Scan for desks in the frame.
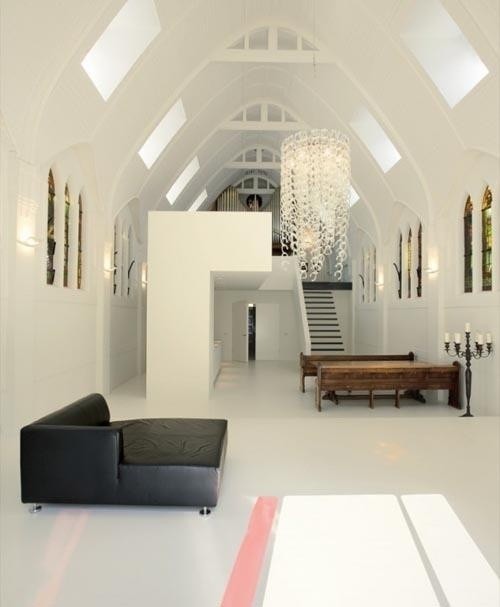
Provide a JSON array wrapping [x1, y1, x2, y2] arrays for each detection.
[[297, 352, 462, 412]]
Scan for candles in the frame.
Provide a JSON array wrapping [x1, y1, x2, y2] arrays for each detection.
[[445, 322, 492, 345]]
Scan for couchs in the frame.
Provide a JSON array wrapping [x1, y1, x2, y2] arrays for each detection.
[[19, 390, 231, 515]]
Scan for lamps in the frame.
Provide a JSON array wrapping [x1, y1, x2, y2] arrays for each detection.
[[276, 0, 355, 282]]
